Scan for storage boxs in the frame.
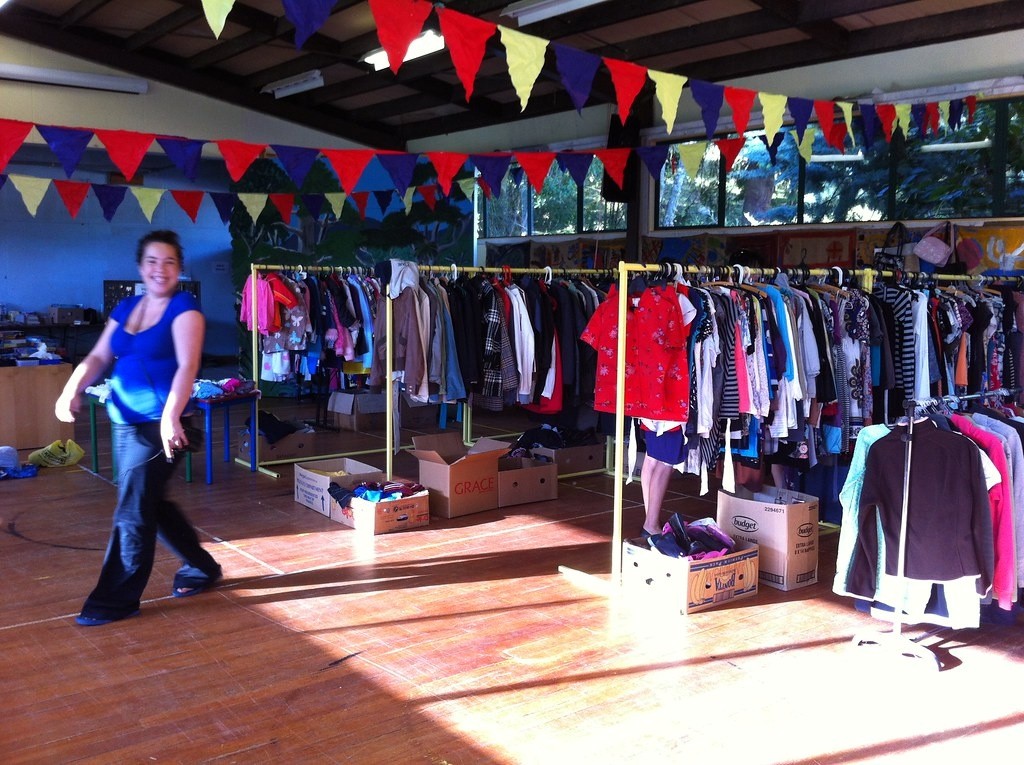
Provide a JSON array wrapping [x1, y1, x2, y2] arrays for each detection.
[[624, 444, 682, 480], [529, 444, 604, 476], [48, 307, 84, 324], [0, 331, 63, 367], [237, 431, 315, 463], [716, 486, 820, 592], [294, 457, 383, 519], [622, 535, 760, 616], [330, 490, 429, 536], [497, 456, 559, 508], [398, 392, 441, 428], [327, 389, 386, 433], [405, 432, 513, 519]]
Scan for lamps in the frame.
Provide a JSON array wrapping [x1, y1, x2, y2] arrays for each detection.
[[810, 153, 865, 162], [0, 63, 149, 96], [921, 139, 994, 152], [259, 70, 324, 100], [499, 0, 607, 28], [358, 27, 445, 73]]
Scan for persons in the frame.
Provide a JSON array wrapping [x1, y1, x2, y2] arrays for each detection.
[[641, 447, 786, 536], [55, 230, 222, 625]]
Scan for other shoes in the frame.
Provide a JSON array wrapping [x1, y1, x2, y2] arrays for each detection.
[[647, 512, 736, 561], [641, 527, 651, 537]]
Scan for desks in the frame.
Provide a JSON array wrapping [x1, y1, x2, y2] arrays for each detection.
[[0, 316, 105, 369], [191, 392, 260, 486], [0, 362, 75, 450], [85, 393, 191, 484]]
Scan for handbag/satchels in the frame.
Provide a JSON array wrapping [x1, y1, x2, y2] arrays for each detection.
[[172, 418, 203, 455], [29, 439, 85, 468]]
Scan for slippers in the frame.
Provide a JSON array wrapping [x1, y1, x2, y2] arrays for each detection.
[[172, 571, 223, 597], [75, 610, 142, 626]]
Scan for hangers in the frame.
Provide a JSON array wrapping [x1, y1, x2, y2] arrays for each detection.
[[626, 263, 1024, 295], [418, 258, 616, 287], [900, 387, 1016, 423], [256, 263, 374, 279]]
